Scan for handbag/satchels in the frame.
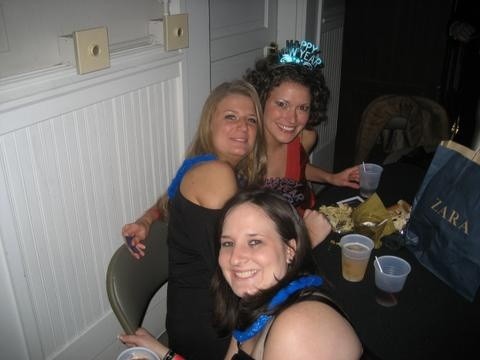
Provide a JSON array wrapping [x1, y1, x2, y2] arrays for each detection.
[[401, 136, 480, 303]]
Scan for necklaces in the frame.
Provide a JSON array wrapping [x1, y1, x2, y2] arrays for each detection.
[[232, 275, 323, 342]]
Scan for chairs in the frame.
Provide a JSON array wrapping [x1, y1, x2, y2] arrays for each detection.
[[355, 95, 448, 166], [106, 219, 169, 348]]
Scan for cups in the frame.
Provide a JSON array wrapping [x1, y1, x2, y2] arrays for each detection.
[[340, 234, 374, 282], [117, 347, 160, 360], [359, 164, 383, 198]]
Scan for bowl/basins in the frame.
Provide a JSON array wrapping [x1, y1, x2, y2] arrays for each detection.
[[373, 256, 411, 292]]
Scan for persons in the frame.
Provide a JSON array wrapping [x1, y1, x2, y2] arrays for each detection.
[[164, 78, 268, 360], [122, 39, 361, 261], [117, 189, 364, 360]]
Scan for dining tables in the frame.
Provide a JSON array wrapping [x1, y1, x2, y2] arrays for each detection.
[[310, 161, 480, 360]]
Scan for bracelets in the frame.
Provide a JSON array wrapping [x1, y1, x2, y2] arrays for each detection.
[[164, 350, 174, 360]]
